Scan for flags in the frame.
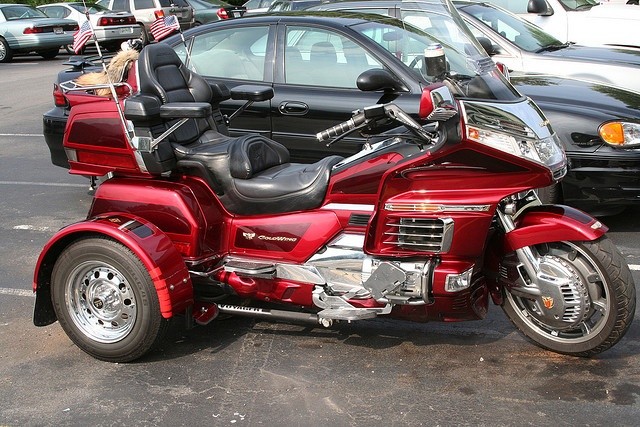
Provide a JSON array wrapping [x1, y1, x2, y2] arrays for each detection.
[[73, 12, 96, 54], [150, 14, 183, 41]]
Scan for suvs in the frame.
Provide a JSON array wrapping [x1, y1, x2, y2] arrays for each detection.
[[85, 0, 196, 48]]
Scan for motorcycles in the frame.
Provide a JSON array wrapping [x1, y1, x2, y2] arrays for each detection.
[[23, 1, 637, 363]]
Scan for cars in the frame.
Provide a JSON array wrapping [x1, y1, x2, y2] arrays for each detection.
[[20, 1, 143, 54], [375, 0, 640, 49], [0, 2, 80, 62], [42, 10, 640, 220], [241, 0, 289, 13], [248, 0, 640, 95], [185, 0, 248, 28]]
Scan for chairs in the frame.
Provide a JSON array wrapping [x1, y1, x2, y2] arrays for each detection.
[[136, 36, 346, 219], [284, 44, 307, 85], [307, 39, 354, 89]]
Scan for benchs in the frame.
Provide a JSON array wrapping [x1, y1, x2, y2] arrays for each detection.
[[195, 48, 265, 83]]
